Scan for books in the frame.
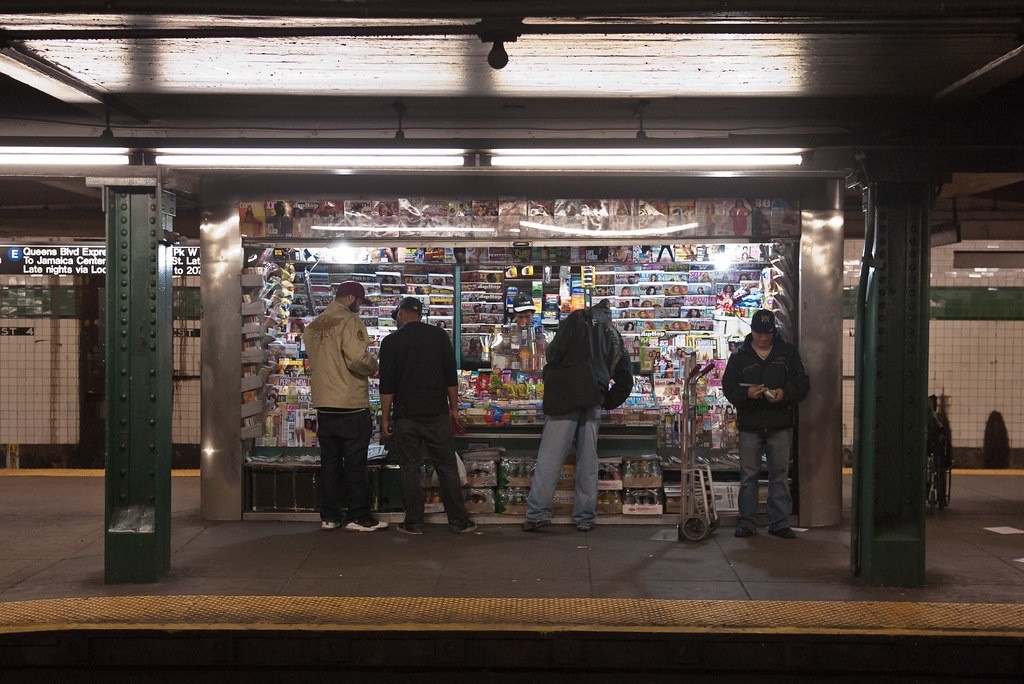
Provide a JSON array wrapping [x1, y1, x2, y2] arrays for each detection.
[[239, 193, 800, 374], [287, 408, 321, 448]]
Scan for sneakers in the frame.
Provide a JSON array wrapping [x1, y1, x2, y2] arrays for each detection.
[[396, 517, 423, 534], [449, 521, 478, 532], [345, 517, 388, 532], [320, 512, 344, 529]]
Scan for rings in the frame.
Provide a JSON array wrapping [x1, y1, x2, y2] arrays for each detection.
[[382, 432, 384, 433]]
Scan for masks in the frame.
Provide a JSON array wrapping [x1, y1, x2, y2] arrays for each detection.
[[348, 297, 360, 313]]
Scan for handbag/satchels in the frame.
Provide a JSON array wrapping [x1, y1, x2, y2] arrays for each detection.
[[542, 362, 600, 416], [431, 450, 468, 488]]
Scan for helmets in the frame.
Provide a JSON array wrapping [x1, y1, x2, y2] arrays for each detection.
[[391, 297, 422, 320]]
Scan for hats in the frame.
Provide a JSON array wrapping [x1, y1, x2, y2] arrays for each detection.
[[593, 299, 612, 319], [335, 281, 373, 304], [513, 292, 537, 312], [751, 309, 775, 333]]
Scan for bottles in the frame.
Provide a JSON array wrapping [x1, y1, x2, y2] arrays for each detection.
[[423, 487, 440, 504], [596, 462, 622, 514], [498, 457, 537, 505], [465, 460, 496, 504], [623, 457, 662, 505], [553, 465, 574, 515]]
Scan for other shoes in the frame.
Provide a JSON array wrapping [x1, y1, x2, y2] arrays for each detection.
[[521, 520, 551, 532], [768, 527, 794, 538], [578, 523, 591, 531], [735, 527, 752, 537]]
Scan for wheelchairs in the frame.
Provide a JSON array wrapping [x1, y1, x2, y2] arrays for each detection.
[[928, 437, 954, 513]]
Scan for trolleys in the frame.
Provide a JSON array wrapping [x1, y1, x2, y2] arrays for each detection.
[[650, 364, 722, 543]]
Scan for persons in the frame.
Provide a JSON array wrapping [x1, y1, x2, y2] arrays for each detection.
[[513, 292, 536, 323], [670, 285, 683, 293], [303, 281, 389, 531], [624, 322, 634, 331], [723, 285, 736, 296], [490, 304, 498, 314], [721, 309, 810, 539], [621, 286, 633, 295], [619, 310, 630, 318], [697, 287, 705, 295], [646, 286, 656, 295], [522, 298, 633, 531], [700, 272, 711, 283], [669, 322, 683, 330], [641, 301, 652, 306], [685, 309, 701, 318], [632, 336, 640, 361], [378, 297, 477, 535]]
